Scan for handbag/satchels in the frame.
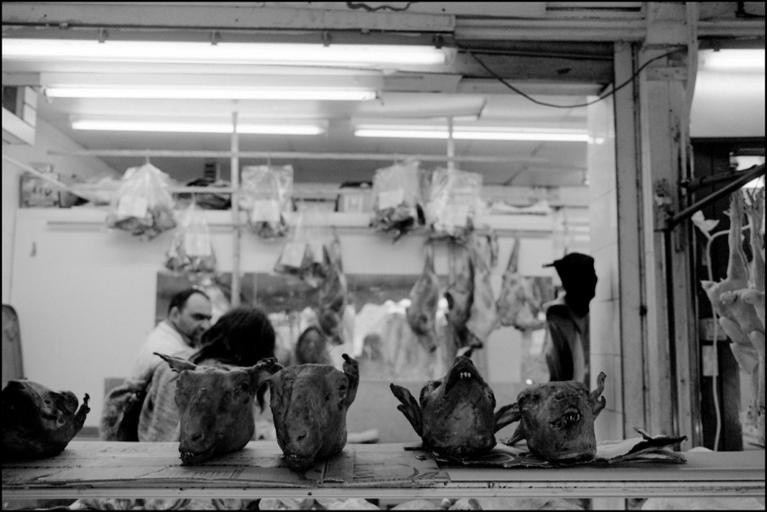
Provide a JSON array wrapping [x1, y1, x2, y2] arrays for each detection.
[[98, 356, 166, 441]]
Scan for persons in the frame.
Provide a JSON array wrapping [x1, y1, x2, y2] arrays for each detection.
[[277, 323, 336, 368], [117, 286, 214, 390], [96, 302, 277, 442], [347, 333, 397, 382], [537, 252, 599, 393]]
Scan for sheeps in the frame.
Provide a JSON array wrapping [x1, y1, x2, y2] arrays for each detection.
[[502, 371, 608, 464], [3, 375, 91, 463], [151, 350, 272, 467], [387, 352, 511, 454], [266, 351, 359, 467]]
